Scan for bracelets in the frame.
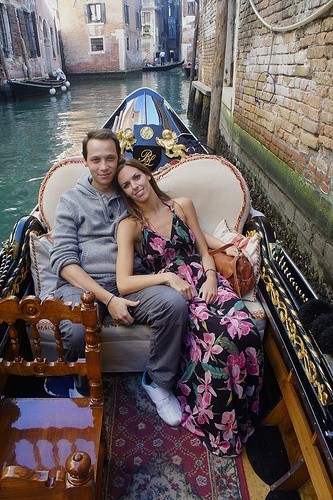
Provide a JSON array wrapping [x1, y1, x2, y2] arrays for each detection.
[[205, 268, 217, 275], [106, 294, 115, 310]]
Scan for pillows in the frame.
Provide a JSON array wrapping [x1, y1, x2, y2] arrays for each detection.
[[211, 219, 262, 302], [28, 229, 57, 296]]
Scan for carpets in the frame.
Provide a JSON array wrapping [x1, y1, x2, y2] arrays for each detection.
[[102, 371, 250, 500]]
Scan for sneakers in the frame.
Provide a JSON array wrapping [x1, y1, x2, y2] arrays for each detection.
[[141, 370, 184, 427]]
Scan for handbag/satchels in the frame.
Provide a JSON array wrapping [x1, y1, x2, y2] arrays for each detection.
[[209, 243, 255, 298]]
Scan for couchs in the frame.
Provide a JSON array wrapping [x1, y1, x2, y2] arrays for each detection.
[[37, 157, 264, 372]]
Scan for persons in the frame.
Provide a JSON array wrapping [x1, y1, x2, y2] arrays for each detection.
[[170, 50, 174, 64], [160, 50, 165, 66], [116, 158, 265, 458], [49, 129, 188, 426]]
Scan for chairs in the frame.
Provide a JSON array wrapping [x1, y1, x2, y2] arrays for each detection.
[[0, 291, 104, 484]]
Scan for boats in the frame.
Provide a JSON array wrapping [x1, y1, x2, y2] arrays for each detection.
[[183, 67, 199, 76], [143, 60, 185, 71], [6, 67, 67, 98]]
